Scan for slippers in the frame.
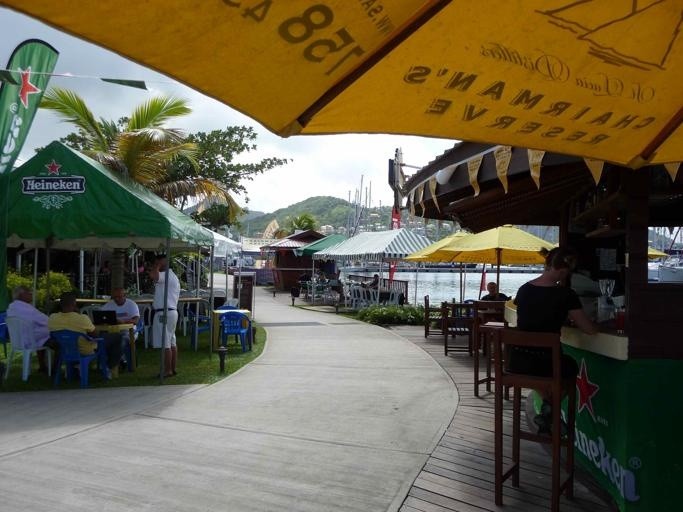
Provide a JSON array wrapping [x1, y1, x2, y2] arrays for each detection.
[[157, 373, 173, 377], [172, 370, 176, 374]]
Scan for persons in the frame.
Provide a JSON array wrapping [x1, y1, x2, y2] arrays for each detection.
[[5, 286, 51, 373], [480, 282, 512, 304], [47, 291, 101, 373], [99, 286, 141, 380], [148, 254, 180, 378], [507, 246, 601, 445]]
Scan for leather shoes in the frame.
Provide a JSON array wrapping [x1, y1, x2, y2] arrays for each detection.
[[52, 365, 63, 376], [39, 367, 49, 376]]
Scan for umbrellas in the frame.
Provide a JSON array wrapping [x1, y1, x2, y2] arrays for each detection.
[[404, 226, 475, 298], [291, 234, 347, 256], [648, 245, 669, 282], [0, 1, 681, 171], [425, 224, 559, 301], [311, 228, 436, 310]]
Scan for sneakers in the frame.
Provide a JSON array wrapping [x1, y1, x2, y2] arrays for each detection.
[[547, 415, 567, 440], [533, 415, 551, 439]]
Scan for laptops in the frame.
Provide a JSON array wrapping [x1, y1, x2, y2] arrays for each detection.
[[92, 310, 121, 325]]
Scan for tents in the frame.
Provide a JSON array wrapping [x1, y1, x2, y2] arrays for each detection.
[[1, 139, 214, 386], [212, 231, 244, 354]]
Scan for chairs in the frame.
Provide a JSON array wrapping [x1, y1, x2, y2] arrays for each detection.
[[80, 306, 102, 321], [389, 289, 400, 306], [219, 312, 251, 353], [48, 329, 109, 387], [472, 299, 521, 399], [424, 295, 451, 338], [221, 298, 239, 307], [217, 305, 239, 345], [442, 302, 474, 356], [188, 310, 211, 347], [4, 317, 50, 381], [136, 303, 153, 348], [179, 289, 226, 337], [348, 286, 378, 308], [492, 329, 574, 510]]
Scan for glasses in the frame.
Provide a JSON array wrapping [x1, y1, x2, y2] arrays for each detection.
[[488, 288, 497, 292]]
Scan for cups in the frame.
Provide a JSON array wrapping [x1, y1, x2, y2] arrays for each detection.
[[598, 280, 616, 313]]
